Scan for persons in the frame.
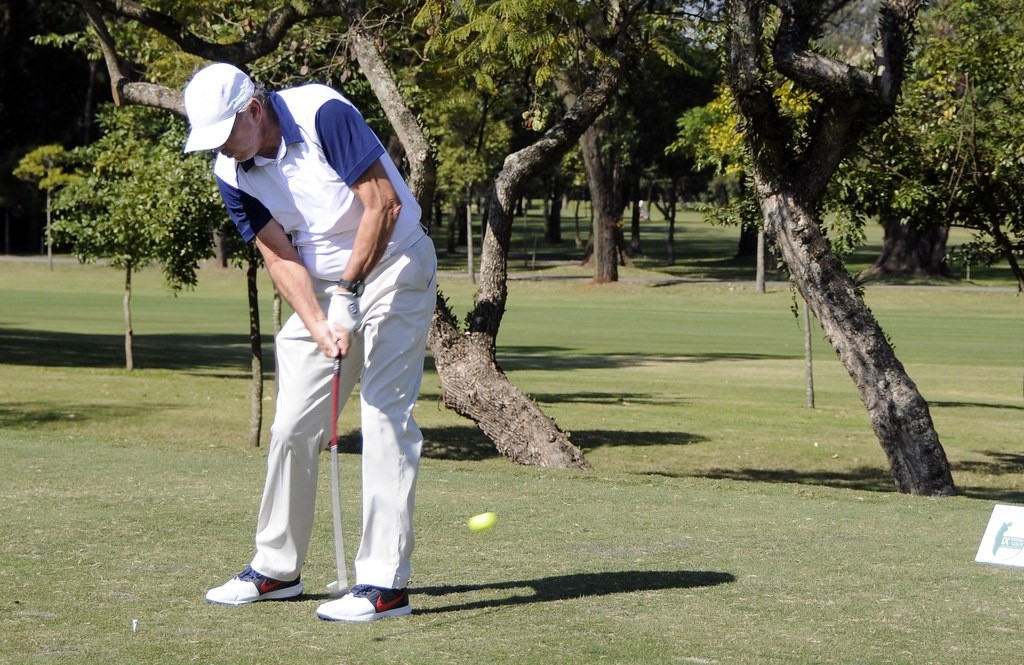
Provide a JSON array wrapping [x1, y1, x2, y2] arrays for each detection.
[[184, 64, 436, 622]]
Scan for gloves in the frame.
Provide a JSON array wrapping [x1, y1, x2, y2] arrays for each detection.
[[328, 292, 360, 342]]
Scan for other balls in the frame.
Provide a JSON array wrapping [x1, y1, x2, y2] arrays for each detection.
[[469, 512, 496, 532]]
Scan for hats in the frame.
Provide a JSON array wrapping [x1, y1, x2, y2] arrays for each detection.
[[184, 63, 255, 153]]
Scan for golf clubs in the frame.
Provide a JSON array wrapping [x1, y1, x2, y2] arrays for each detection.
[[327, 342, 349, 597]]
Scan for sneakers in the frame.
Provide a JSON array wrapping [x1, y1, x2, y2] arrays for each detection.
[[316, 584, 412, 621], [206, 566, 303, 605]]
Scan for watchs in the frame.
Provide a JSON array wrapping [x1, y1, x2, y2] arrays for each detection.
[[338, 279, 365, 297]]
